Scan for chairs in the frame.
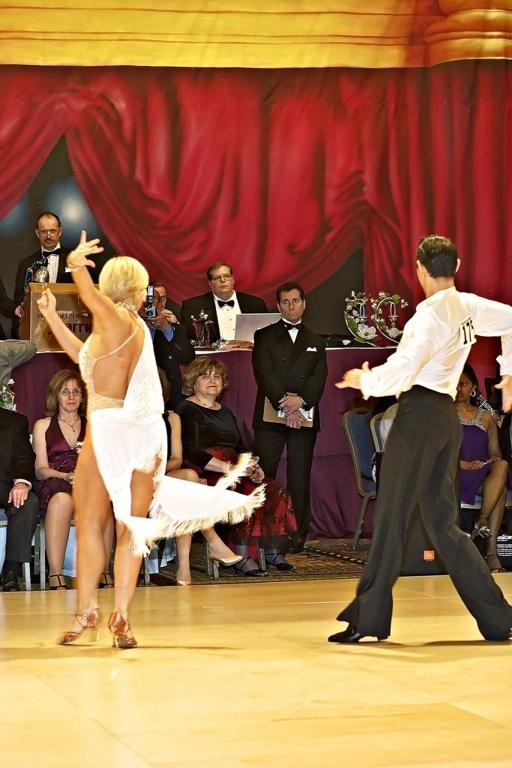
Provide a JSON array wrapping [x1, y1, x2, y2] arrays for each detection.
[[203, 535, 266, 580], [371, 412, 385, 451], [0, 520, 31, 590], [143, 540, 219, 584], [343, 408, 377, 548]]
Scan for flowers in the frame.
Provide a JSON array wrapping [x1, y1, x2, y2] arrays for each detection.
[[345, 291, 408, 343]]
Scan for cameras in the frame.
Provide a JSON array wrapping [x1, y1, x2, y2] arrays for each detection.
[[144, 284, 156, 318]]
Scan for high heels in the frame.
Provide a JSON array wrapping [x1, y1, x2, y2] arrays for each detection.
[[208, 544, 244, 567], [107, 611, 137, 648], [57, 607, 102, 645], [468, 522, 494, 540]]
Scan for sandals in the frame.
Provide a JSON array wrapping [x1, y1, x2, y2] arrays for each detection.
[[232, 557, 270, 577], [483, 552, 507, 573], [266, 552, 299, 573], [98, 571, 115, 589], [46, 573, 69, 590]]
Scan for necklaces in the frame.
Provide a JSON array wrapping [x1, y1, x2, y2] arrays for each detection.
[[59, 415, 79, 432]]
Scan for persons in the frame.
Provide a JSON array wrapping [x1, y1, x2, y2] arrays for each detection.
[[1, 282, 24, 340], [138, 282, 298, 587], [10, 213, 74, 340], [37, 230, 267, 648], [328, 234, 512, 644], [252, 282, 328, 554], [1, 408, 40, 591], [33, 369, 114, 590], [456, 363, 509, 572], [180, 261, 269, 351]]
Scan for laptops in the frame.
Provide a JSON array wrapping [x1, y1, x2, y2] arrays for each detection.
[[234, 313, 281, 344]]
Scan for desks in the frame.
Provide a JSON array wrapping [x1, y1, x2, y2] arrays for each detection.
[[12, 347, 399, 541]]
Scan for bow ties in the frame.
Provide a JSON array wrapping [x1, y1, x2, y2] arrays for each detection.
[[283, 322, 304, 330], [218, 300, 234, 308], [43, 248, 62, 258]]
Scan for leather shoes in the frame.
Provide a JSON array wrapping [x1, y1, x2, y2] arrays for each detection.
[[174, 566, 193, 586], [0, 570, 21, 592], [327, 622, 389, 645]]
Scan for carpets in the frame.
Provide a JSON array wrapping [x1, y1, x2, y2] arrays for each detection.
[[17, 537, 370, 591]]
